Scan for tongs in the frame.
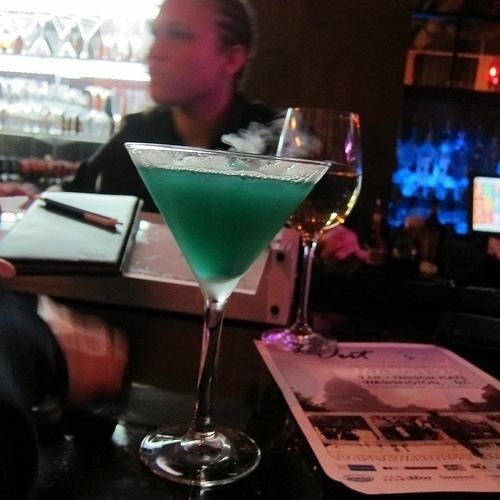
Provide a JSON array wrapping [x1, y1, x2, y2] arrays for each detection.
[[124, 142, 331, 487]]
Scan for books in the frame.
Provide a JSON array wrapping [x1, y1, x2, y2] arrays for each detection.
[[0, 191, 144, 277]]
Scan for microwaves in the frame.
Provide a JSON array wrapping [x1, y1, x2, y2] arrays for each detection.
[[403, 49, 500, 92]]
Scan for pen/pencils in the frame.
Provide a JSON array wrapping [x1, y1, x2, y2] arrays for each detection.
[[33, 194, 122, 228]]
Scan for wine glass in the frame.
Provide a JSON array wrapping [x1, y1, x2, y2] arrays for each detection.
[[261, 105, 364, 354]]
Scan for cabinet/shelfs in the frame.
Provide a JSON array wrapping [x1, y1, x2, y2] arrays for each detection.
[[0, 1, 153, 145]]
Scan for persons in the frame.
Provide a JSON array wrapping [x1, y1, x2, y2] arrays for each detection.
[[1, 0, 372, 264], [1, 260, 129, 500]]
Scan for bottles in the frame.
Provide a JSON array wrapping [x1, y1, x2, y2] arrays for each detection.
[[0, 14, 153, 190], [371, 110, 500, 272]]
